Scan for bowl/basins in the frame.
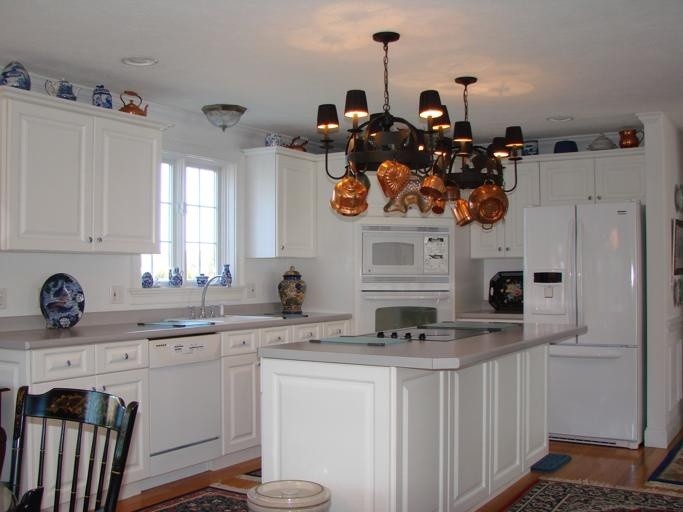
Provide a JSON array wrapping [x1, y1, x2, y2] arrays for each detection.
[[588, 134, 615, 151]]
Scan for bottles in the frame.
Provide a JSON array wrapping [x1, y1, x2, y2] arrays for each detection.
[[219, 264, 232, 287], [265, 131, 282, 147], [92, 83, 113, 110], [0, 59, 32, 91], [278, 265, 308, 314]]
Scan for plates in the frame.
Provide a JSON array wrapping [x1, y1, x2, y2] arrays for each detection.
[[489, 271, 523, 314], [39, 272, 87, 329]]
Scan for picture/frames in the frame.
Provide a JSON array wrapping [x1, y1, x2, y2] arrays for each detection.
[[673, 219, 683, 276]]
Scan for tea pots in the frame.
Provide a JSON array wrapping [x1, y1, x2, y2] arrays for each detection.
[[43, 77, 81, 103], [617, 128, 644, 148], [167, 267, 185, 288], [438, 180, 461, 201], [117, 89, 149, 118], [280, 135, 310, 153], [329, 175, 369, 217], [420, 175, 445, 198]]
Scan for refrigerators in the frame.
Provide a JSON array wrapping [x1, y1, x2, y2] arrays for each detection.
[[520, 200, 646, 451]]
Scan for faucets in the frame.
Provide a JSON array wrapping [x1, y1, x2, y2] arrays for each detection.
[[200, 275, 231, 318]]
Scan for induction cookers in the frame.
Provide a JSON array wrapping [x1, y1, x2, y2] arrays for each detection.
[[351, 321, 500, 343]]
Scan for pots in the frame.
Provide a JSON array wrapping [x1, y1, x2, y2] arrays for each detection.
[[468, 180, 510, 230]]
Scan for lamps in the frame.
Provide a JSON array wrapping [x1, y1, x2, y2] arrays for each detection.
[[316, 31, 451, 216], [435, 75, 524, 229], [202, 104, 246, 131]]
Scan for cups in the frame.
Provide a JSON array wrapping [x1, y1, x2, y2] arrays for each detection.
[[195, 274, 210, 287], [432, 199, 446, 214]]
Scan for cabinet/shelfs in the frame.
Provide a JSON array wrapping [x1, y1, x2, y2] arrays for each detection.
[[291, 320, 350, 343], [470, 154, 540, 258], [0, 85, 160, 254], [540, 146, 646, 207], [221, 325, 291, 470], [242, 147, 317, 257], [0, 338, 149, 512]]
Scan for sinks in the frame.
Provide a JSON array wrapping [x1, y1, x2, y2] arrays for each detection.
[[164, 315, 285, 326]]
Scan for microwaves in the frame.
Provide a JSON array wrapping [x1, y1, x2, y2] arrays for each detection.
[[358, 223, 450, 284]]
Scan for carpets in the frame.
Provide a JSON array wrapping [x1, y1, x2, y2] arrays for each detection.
[[645, 439, 683, 490], [236, 468, 261, 482], [134, 485, 251, 512], [498, 476, 683, 512]]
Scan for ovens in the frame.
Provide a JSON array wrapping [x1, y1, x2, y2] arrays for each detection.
[[358, 289, 452, 335]]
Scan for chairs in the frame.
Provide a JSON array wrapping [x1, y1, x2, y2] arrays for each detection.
[[9, 385, 138, 512]]
